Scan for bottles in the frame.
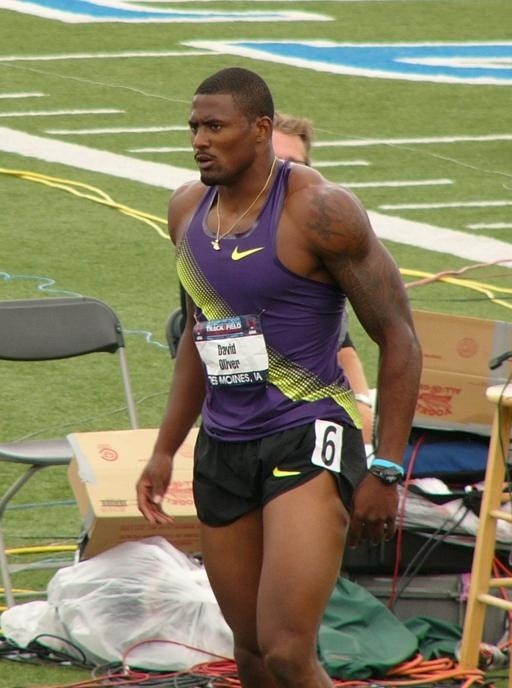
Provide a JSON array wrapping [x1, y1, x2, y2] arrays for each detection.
[[455, 639, 509, 671]]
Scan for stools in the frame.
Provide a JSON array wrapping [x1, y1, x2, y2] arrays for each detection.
[[459, 384, 511, 669]]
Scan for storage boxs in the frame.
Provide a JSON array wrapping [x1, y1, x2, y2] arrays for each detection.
[[66, 427, 204, 564], [410, 309, 511, 439]]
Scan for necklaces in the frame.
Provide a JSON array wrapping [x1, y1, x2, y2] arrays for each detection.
[[208, 153, 280, 254]]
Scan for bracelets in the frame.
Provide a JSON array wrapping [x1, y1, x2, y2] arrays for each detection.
[[355, 394, 374, 411], [364, 456, 406, 487]]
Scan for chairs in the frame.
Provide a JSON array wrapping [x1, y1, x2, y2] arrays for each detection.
[[0, 298, 138, 608]]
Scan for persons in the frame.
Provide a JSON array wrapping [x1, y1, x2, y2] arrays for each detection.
[[268, 109, 376, 485], [134, 64, 424, 687]]
